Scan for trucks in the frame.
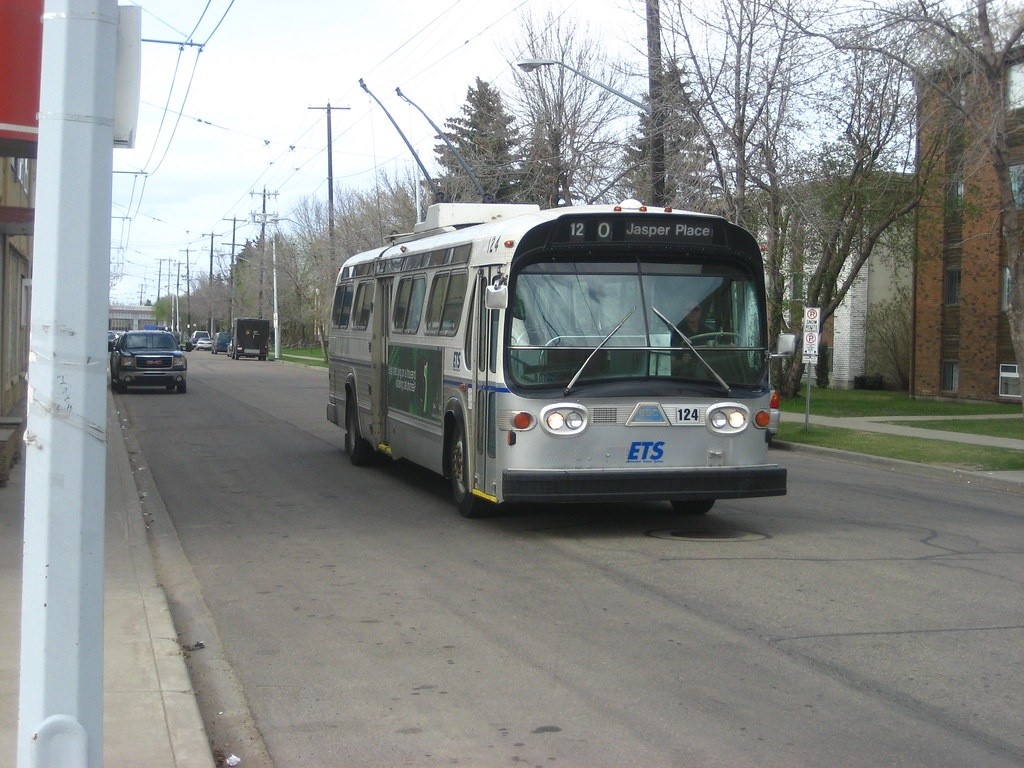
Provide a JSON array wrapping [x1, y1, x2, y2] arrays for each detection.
[[233, 318, 268, 361]]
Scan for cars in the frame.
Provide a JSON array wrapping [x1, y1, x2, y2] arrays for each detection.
[[108, 330, 127, 352], [188, 328, 233, 357]]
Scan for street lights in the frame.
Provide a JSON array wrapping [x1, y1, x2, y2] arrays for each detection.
[[271, 217, 337, 290], [516, 56, 666, 211], [219, 253, 266, 319]]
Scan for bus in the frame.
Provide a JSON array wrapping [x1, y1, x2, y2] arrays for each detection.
[[326, 200, 791, 519]]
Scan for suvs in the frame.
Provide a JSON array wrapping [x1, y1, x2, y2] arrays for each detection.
[[110, 330, 187, 393]]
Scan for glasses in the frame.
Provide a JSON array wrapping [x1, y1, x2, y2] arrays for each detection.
[[685, 306, 700, 313]]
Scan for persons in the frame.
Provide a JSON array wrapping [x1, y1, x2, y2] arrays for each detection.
[[665, 298, 736, 367]]
